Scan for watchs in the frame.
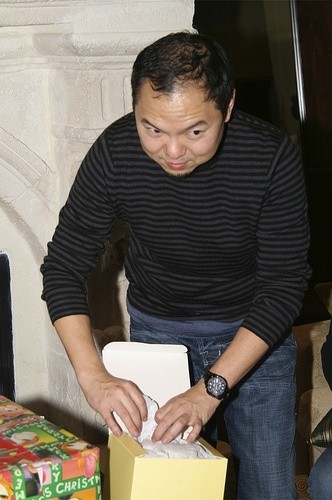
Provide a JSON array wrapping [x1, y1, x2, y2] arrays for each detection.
[[201, 370, 232, 403]]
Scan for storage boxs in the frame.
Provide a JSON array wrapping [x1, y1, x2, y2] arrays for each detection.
[[101, 341, 229, 499]]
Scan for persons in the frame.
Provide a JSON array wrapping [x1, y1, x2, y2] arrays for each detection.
[[36, 27, 313, 500], [305, 320, 332, 500]]
